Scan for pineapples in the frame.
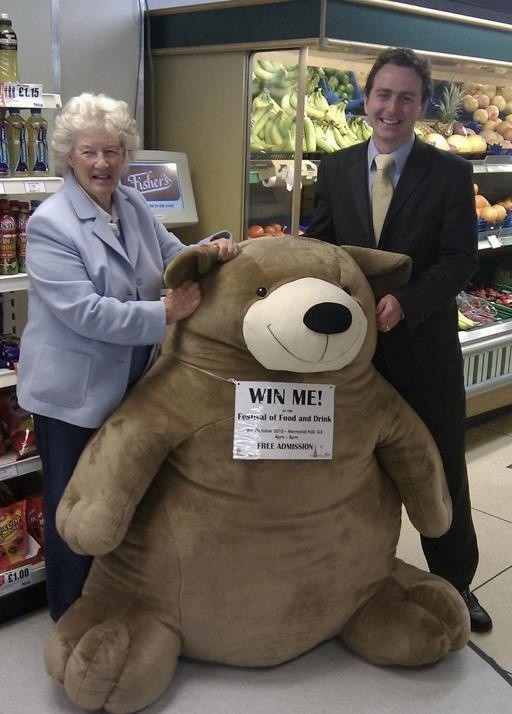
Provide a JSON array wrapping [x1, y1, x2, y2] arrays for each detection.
[[415, 82, 465, 135]]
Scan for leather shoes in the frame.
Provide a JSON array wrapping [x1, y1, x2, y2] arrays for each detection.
[[455, 585, 492, 632]]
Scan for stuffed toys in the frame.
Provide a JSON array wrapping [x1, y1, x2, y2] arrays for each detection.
[[42, 239, 471, 714]]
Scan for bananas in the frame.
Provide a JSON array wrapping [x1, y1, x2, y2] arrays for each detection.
[[457, 309, 474, 331], [248, 59, 372, 155]]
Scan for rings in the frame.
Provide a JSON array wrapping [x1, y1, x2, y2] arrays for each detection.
[[386, 326, 391, 332]]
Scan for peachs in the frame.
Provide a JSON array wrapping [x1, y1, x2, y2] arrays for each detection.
[[460, 83, 512, 149]]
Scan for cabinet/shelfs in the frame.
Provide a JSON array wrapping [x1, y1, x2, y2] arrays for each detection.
[[0, 93, 68, 597], [142, 0, 512, 420]]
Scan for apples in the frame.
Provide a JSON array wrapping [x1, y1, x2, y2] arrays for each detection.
[[424, 131, 487, 152], [247, 222, 300, 240], [473, 184, 511, 222]]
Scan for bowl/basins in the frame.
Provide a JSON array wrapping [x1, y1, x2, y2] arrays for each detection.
[[461, 295, 497, 317]]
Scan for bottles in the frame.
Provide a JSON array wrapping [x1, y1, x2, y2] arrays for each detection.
[[1, 11, 21, 85], [0, 196, 43, 276], [0, 106, 49, 179]]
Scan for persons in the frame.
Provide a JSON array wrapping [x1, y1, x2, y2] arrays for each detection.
[[17, 91, 241, 621], [308, 48, 493, 632]]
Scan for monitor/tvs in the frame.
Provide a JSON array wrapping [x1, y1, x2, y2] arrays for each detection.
[[118, 149, 199, 230]]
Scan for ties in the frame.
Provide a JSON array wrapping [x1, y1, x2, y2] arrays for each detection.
[[371, 154, 395, 247]]
